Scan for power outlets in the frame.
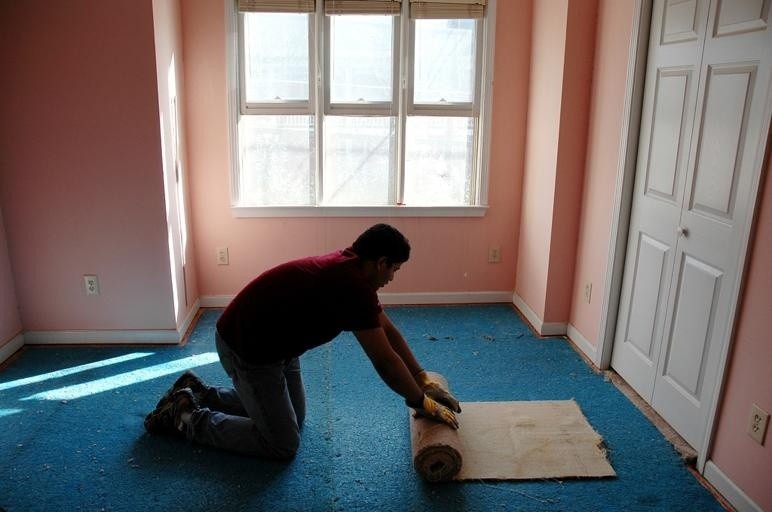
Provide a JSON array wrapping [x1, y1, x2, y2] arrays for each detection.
[[85, 276, 99, 295], [746, 403, 770, 444]]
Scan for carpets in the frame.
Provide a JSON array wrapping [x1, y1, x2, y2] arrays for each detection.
[[409, 399, 618, 479]]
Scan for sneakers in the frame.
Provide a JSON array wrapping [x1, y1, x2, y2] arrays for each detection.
[[144, 387, 198, 436], [156, 369, 210, 408]]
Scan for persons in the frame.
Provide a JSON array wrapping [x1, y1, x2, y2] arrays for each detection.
[[144, 223, 462, 460]]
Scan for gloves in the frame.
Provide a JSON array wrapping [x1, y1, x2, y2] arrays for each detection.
[[411, 392, 459, 430], [413, 369, 461, 413]]
